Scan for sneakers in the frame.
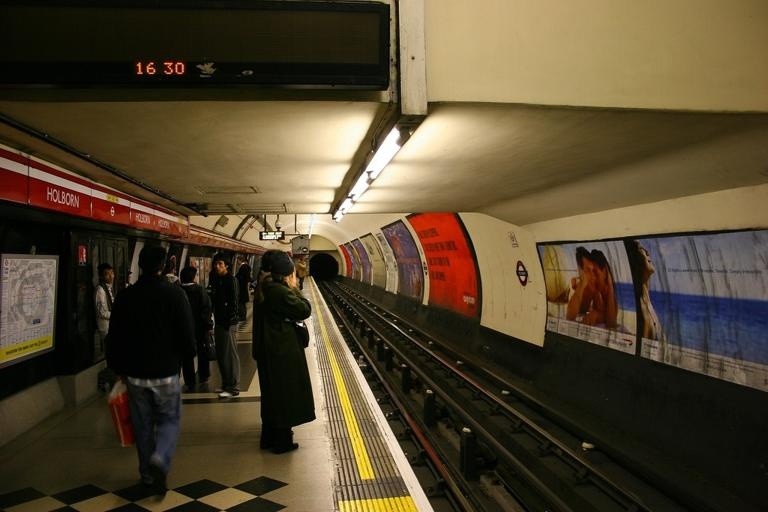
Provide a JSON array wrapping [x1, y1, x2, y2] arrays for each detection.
[[214, 388, 234, 397]]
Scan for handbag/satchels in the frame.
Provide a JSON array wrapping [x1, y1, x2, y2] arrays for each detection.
[[200, 334, 216, 360], [107, 381, 135, 448], [293, 320, 309, 348]]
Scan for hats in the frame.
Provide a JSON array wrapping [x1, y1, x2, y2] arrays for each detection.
[[270, 251, 293, 276]]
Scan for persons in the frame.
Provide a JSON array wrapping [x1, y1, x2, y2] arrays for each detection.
[[582, 249, 618, 328], [623, 239, 663, 339], [565, 246, 604, 326], [258, 258, 317, 455], [106, 243, 197, 497], [251, 248, 295, 438], [93, 247, 308, 405]]
